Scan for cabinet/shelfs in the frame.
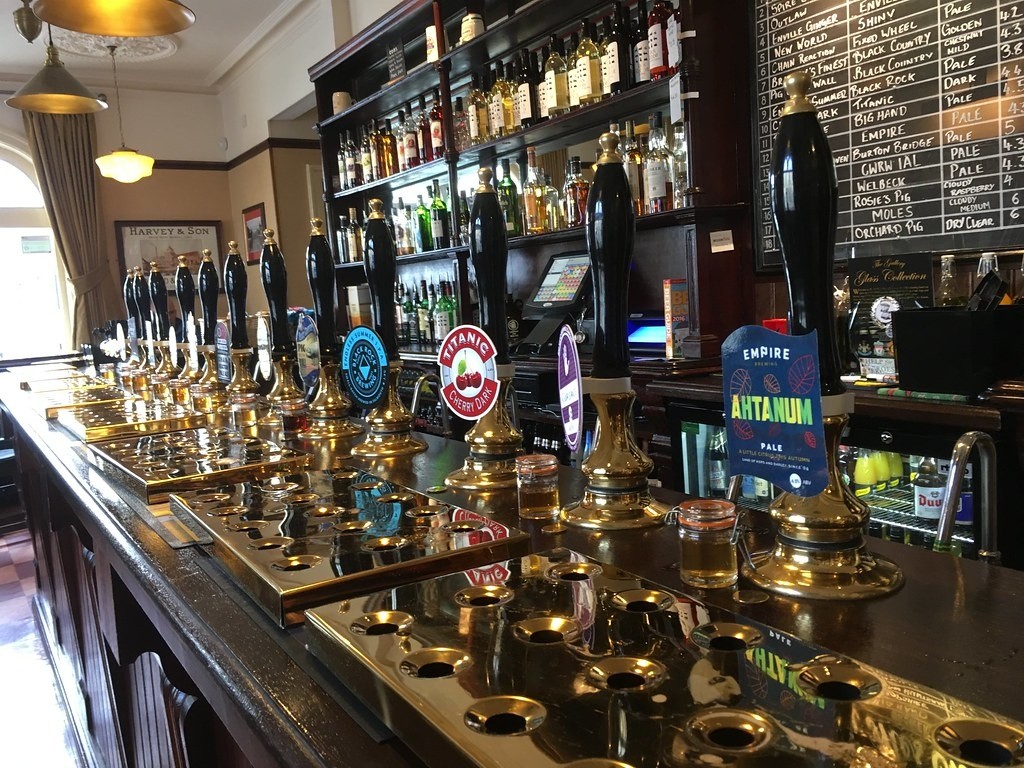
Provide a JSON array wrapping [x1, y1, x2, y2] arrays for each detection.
[[306, 0, 751, 381], [647, 372, 1001, 565]]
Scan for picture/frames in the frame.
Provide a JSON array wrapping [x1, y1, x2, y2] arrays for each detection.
[[242, 202, 266, 267], [113, 218, 225, 296]]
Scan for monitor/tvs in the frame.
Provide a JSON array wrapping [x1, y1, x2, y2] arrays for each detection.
[[523, 250, 596, 320]]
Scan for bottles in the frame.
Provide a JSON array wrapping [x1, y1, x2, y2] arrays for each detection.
[[334, 130, 361, 189], [499, 147, 588, 239], [609, 1, 684, 97], [932, 252, 1013, 306], [492, 49, 545, 139], [355, 119, 396, 184], [836, 444, 903, 493], [607, 112, 690, 216], [393, 280, 458, 345], [547, 18, 610, 118], [392, 198, 413, 258], [710, 425, 777, 501], [458, 0, 487, 47], [453, 62, 489, 152], [910, 453, 975, 523], [397, 89, 444, 173], [335, 208, 366, 263], [416, 180, 451, 253]]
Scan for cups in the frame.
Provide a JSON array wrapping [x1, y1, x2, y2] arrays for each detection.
[[120, 366, 131, 385], [151, 373, 169, 400], [101, 363, 116, 382], [192, 384, 212, 409], [676, 499, 736, 589], [168, 379, 190, 404], [280, 398, 304, 439], [230, 394, 258, 425], [129, 368, 150, 391], [332, 92, 357, 115], [517, 455, 562, 516]]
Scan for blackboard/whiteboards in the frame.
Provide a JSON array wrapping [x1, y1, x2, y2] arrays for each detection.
[[752, 0, 1017, 277]]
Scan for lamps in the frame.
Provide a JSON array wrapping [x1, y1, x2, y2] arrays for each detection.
[[13, 0, 43, 44], [94, 46, 155, 184], [32, 0, 197, 37], [4, 24, 108, 115]]
